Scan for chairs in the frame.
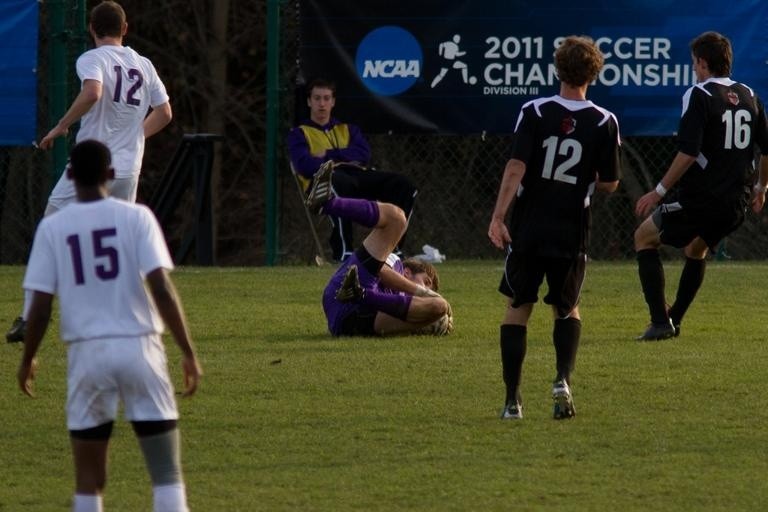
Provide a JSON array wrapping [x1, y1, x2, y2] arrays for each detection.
[[283, 156, 339, 265]]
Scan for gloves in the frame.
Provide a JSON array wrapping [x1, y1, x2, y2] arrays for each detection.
[[414, 283, 452, 335]]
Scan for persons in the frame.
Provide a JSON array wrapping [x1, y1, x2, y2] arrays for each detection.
[[633, 31, 768, 339], [6, 1, 172, 341], [16, 140, 204, 511], [304, 156, 453, 338], [283, 79, 419, 263], [487, 36, 622, 420]]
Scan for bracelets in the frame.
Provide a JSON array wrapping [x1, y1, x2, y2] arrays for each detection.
[[754, 180, 768, 194], [654, 182, 668, 197]]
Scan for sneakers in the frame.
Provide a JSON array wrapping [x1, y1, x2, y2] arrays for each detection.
[[335, 265, 363, 304], [5, 316, 29, 345], [302, 159, 338, 212], [551, 378, 583, 418], [634, 315, 681, 342], [501, 395, 524, 420]]
